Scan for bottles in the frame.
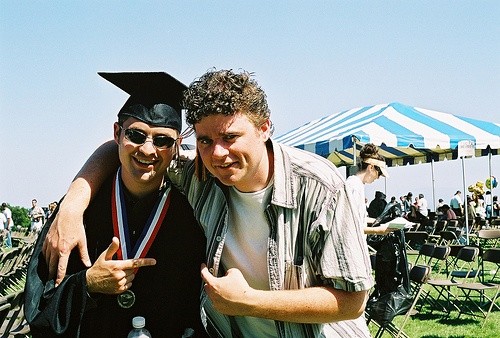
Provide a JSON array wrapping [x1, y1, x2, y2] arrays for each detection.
[[127, 316, 151, 338]]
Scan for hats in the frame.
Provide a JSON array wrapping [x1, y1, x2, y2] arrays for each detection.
[[97, 72, 188, 135], [363, 158, 387, 177]]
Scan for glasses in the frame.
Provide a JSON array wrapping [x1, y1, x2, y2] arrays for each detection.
[[120, 126, 178, 149]]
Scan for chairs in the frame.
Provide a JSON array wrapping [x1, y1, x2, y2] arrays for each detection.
[[0, 238, 37, 338], [365, 211, 500, 338]]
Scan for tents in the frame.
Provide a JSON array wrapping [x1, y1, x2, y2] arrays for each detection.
[[271, 101, 500, 247]]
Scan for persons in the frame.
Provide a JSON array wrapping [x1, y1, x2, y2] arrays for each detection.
[[0, 202, 14, 249], [344, 143, 399, 277], [364, 189, 500, 228], [23, 72, 207, 338], [40, 67, 376, 338], [27, 199, 56, 232]]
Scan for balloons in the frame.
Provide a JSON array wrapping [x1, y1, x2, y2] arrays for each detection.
[[468, 181, 485, 196], [486, 175, 498, 190]]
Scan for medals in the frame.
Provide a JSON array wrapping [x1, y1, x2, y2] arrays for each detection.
[[117, 290, 136, 308]]
